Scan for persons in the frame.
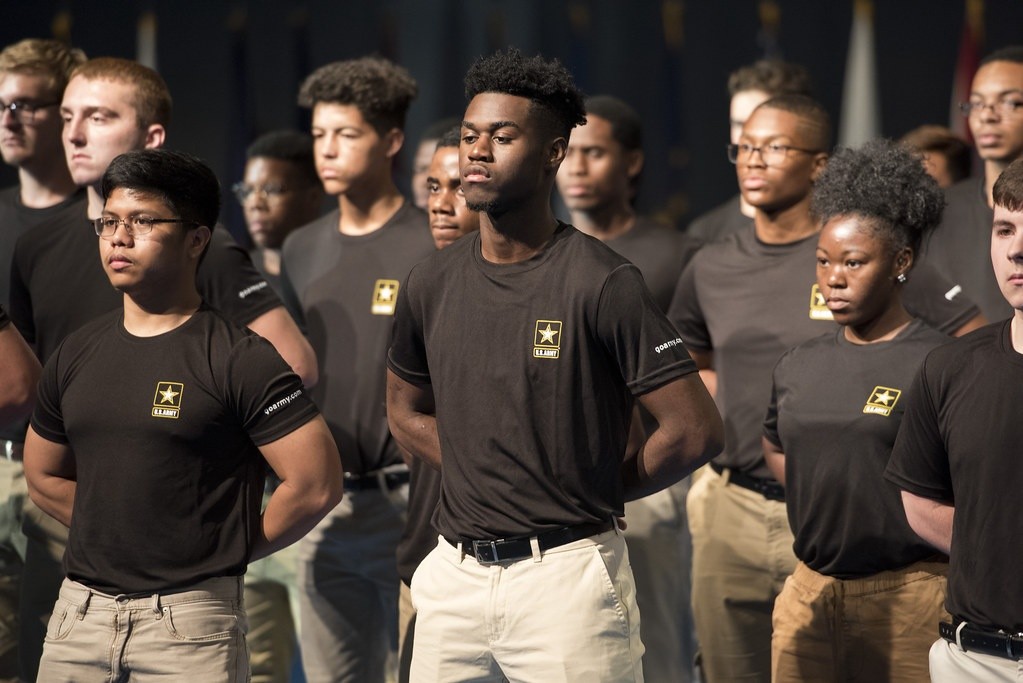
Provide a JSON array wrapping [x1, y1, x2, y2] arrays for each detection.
[[761, 138, 961, 682], [23, 148, 342, 682], [0, 39, 1023, 683], [383, 51, 725, 683], [882, 155, 1023, 683]]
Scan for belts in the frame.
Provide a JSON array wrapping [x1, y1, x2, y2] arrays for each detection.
[[345, 471, 410, 491], [938, 617, 1023, 660], [445, 520, 628, 564], [709, 464, 785, 501]]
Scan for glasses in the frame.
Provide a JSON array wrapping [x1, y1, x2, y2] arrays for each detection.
[[961, 99, 1023, 117], [0, 101, 61, 118], [728, 142, 819, 166], [95, 213, 194, 236], [237, 185, 306, 209]]
